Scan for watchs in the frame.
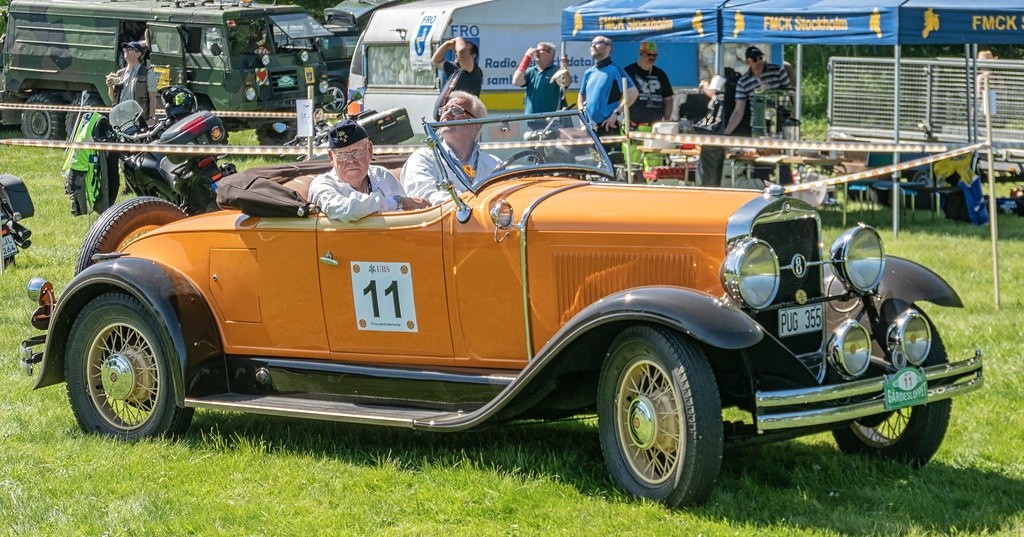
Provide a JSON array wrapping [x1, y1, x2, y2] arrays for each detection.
[[393, 195, 403, 209]]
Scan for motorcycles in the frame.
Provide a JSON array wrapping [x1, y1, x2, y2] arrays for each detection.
[[105, 85, 237, 217], [0, 173, 34, 269], [273, 79, 414, 163]]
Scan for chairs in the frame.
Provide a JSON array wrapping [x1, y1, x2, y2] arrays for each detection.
[[844, 152, 944, 227]]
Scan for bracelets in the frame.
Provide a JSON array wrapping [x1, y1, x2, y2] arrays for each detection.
[[614, 110, 620, 116]]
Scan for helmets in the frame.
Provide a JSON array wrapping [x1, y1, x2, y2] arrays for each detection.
[[162, 85, 198, 118]]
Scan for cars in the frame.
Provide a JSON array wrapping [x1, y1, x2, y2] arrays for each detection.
[[22, 107, 985, 511]]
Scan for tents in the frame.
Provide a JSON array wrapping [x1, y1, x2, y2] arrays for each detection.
[[559, 0, 1024, 239]]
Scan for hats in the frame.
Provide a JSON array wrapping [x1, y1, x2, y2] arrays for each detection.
[[746, 46, 764, 58], [640, 41, 658, 52], [328, 120, 370, 150], [121, 40, 144, 56]]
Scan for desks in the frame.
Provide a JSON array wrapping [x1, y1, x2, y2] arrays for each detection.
[[636, 147, 822, 189]]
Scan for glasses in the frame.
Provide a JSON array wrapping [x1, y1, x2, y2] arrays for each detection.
[[749, 57, 764, 62], [123, 48, 135, 52], [589, 40, 609, 47], [439, 105, 478, 119], [643, 51, 658, 57], [331, 141, 367, 161], [537, 48, 551, 55]]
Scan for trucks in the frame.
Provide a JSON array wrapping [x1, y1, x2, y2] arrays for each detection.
[[344, 0, 785, 134], [0, 1, 397, 147]]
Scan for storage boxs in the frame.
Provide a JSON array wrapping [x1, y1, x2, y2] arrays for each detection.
[[356, 107, 414, 145], [158, 110, 228, 165]]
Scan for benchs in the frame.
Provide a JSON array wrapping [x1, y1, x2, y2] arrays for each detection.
[[283, 167, 402, 201]]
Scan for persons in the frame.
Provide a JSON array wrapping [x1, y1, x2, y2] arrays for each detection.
[[512, 42, 573, 132], [400, 90, 502, 207], [577, 36, 639, 153], [307, 118, 433, 223], [431, 36, 483, 122], [977, 50, 1008, 129], [724, 45, 795, 186], [105, 41, 157, 126], [621, 42, 673, 184]]
